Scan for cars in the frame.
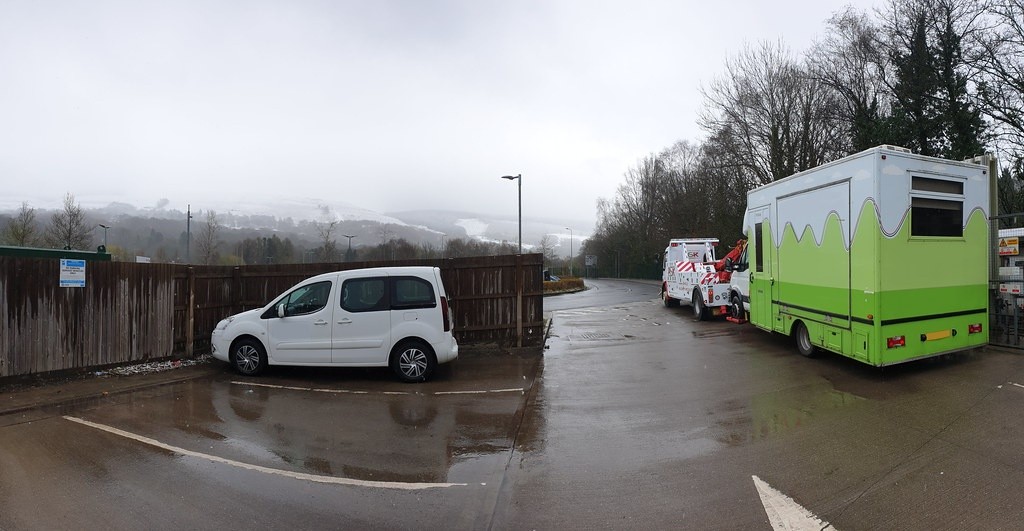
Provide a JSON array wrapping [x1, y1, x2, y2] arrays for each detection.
[[210, 265, 459, 384]]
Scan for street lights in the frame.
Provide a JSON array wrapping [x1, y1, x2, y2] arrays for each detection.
[[97, 222, 112, 252], [501, 173, 522, 255], [565, 228, 572, 278]]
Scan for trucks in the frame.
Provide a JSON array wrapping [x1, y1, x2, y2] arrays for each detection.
[[660, 238, 730, 322], [728, 145, 990, 368]]
